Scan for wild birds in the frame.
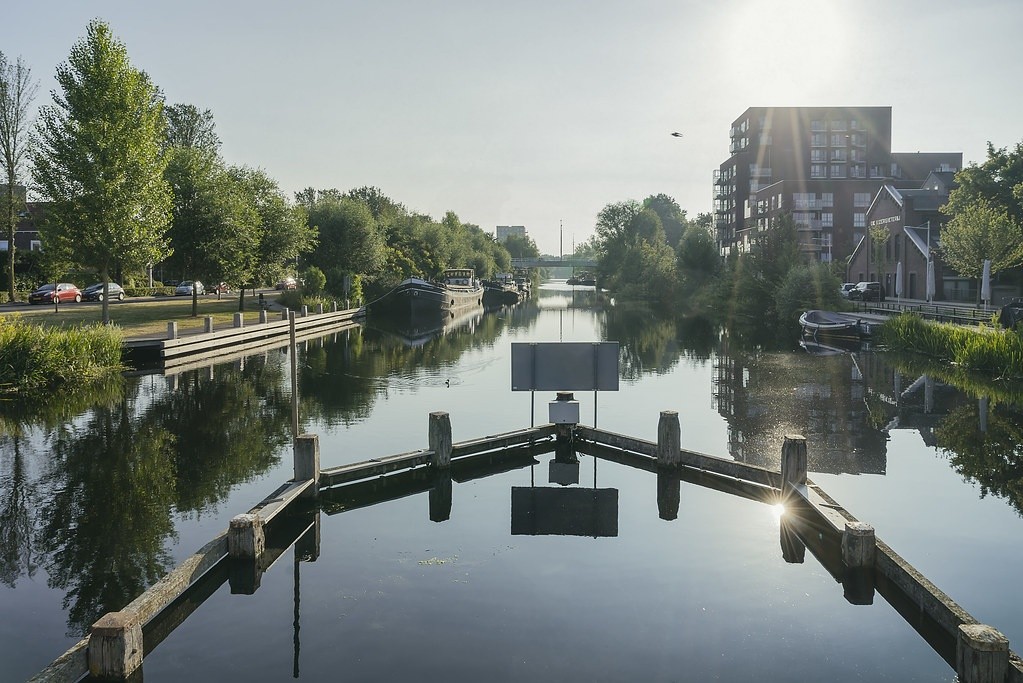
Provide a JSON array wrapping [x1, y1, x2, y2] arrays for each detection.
[[670, 131, 684, 138], [444, 378, 451, 388]]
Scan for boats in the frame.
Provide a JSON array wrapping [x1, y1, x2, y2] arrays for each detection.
[[393, 267, 484, 313], [798, 309, 863, 348]]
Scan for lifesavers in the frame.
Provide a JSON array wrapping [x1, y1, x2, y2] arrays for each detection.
[[443, 279, 450, 284], [462, 278, 469, 285]]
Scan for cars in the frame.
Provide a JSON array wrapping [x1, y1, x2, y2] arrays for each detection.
[[175, 280, 206, 296], [849, 282, 886, 301], [840, 283, 855, 299], [80, 282, 125, 302], [211, 282, 230, 295], [28, 283, 82, 305], [275, 278, 297, 290]]
